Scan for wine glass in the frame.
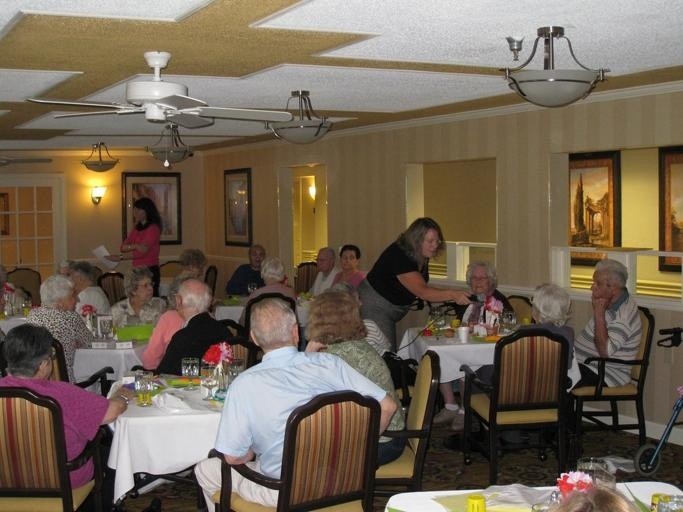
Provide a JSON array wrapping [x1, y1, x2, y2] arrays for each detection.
[[100, 319, 114, 341], [181, 357, 200, 390], [430, 303, 446, 332], [247, 282, 257, 296], [201, 364, 220, 401], [135, 371, 153, 406]]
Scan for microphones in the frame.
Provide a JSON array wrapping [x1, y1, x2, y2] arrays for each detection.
[[446, 293, 487, 304]]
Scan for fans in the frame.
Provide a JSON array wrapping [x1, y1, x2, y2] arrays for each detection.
[[25, 51, 293, 123], [0, 154, 54, 167]]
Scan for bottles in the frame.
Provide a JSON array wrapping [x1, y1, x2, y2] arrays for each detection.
[[466, 495, 487, 512], [652, 494, 670, 512]]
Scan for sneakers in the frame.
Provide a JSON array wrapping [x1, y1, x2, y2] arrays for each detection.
[[432, 404, 462, 423], [450, 409, 464, 432]]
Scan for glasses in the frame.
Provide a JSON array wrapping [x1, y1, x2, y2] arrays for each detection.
[[134, 281, 156, 289]]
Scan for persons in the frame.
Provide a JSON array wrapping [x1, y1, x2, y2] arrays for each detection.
[[1, 196, 642, 511]]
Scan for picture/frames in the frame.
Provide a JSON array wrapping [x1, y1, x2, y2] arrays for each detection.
[[569, 150, 622, 266], [121, 171, 183, 245], [223, 168, 252, 246], [657, 147, 682, 271], [0, 192, 9, 235]]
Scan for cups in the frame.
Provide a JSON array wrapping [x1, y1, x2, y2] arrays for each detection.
[[502, 311, 517, 329], [576, 456, 608, 488], [657, 495, 683, 512], [310, 287, 321, 297], [222, 359, 246, 391], [485, 326, 500, 336], [532, 500, 554, 512], [127, 314, 141, 326], [443, 329, 455, 338], [458, 327, 470, 344]]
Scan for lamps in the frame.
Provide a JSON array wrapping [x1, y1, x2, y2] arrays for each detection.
[[265, 90, 334, 144], [90, 187, 103, 205], [145, 123, 195, 168], [80, 141, 120, 172], [499, 26, 610, 108]]
